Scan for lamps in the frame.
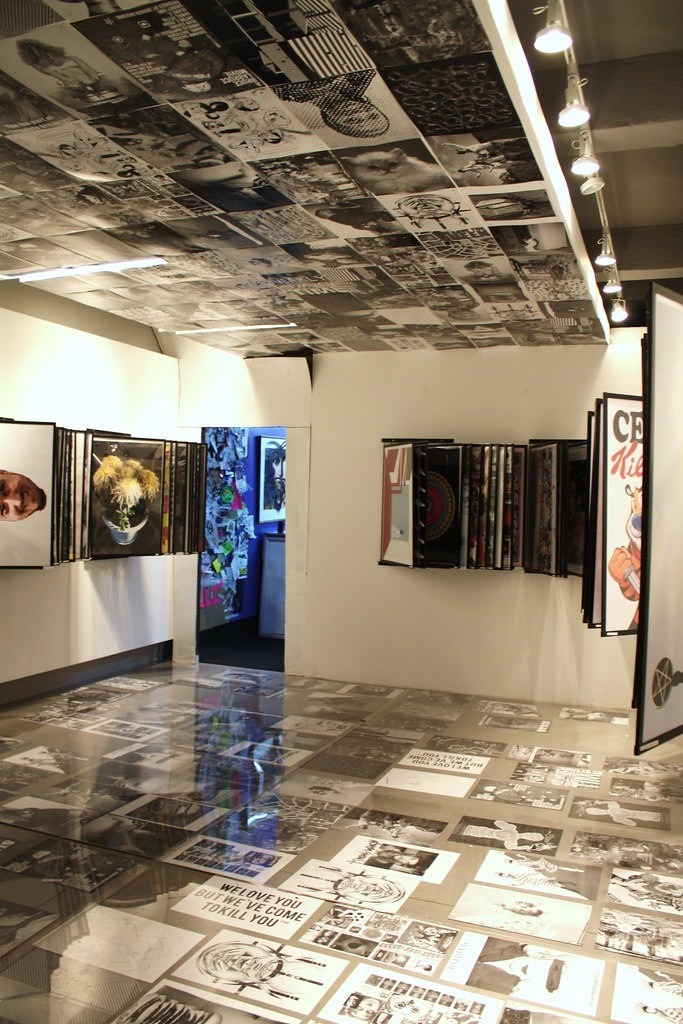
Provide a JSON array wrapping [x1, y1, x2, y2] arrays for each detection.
[[535, 0, 628, 324]]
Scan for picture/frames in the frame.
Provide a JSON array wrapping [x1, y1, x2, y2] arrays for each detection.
[[0, 419, 205, 569], [380, 281, 683, 755], [257, 435, 286, 524]]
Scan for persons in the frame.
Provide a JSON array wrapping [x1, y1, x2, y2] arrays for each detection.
[[0, 469, 46, 522]]
